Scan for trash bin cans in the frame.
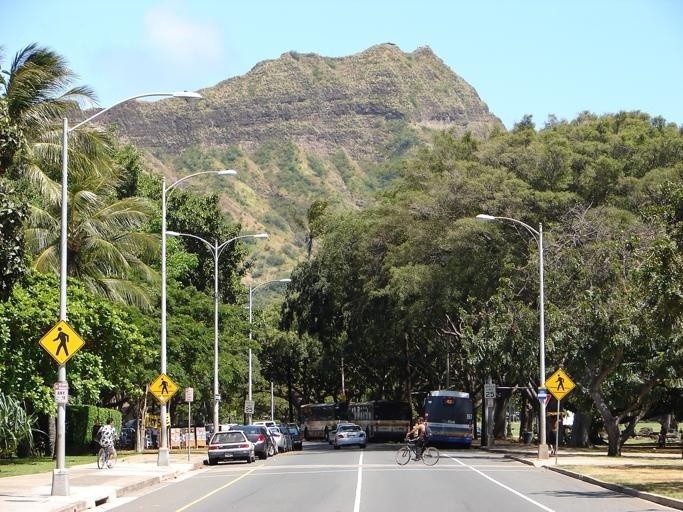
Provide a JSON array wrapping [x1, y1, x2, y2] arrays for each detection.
[[523, 432, 533, 443]]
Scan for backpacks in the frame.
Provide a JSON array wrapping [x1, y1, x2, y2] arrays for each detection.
[[422, 423, 432, 438]]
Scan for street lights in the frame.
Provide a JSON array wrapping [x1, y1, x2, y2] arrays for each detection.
[[245, 277, 292, 423], [50, 88, 204, 499], [157, 168, 238, 465], [165, 228, 269, 433], [476, 212, 551, 459]]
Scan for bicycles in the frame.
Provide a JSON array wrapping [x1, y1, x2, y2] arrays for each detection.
[[395, 434, 439, 466], [94, 434, 117, 470]]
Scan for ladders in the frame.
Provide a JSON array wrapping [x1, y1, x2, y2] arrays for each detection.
[[146, 430, 152, 449]]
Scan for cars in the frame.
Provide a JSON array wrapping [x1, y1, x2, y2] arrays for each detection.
[[335, 425, 366, 448], [253, 421, 302, 453], [476, 427, 480, 437], [505, 410, 519, 422], [208, 430, 255, 463]]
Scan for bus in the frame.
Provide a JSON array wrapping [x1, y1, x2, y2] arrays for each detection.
[[419, 390, 473, 449], [350, 401, 411, 441], [302, 402, 348, 440]]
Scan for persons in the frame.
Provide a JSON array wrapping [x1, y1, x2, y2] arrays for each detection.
[[95, 417, 117, 466], [158, 378, 169, 394], [180, 431, 183, 447], [555, 374, 565, 392], [406, 417, 429, 459], [411, 419, 419, 440], [323, 424, 328, 437], [50, 327, 69, 356]]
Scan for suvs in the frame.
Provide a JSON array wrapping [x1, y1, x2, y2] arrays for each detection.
[[228, 425, 275, 461], [327, 422, 354, 445]]
